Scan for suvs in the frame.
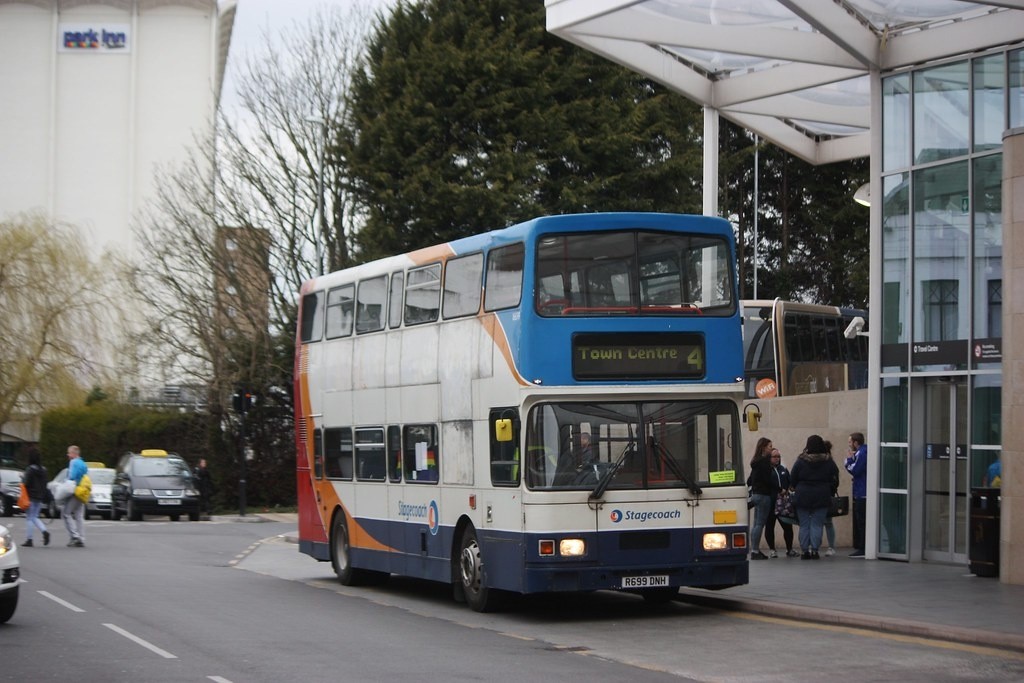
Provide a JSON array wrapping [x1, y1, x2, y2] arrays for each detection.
[[109, 450, 201, 522]]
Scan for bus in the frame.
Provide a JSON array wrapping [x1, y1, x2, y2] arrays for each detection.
[[293, 211, 765, 613], [671, 297, 869, 400]]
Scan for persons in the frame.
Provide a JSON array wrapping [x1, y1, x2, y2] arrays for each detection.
[[746, 437, 775, 560], [193, 458, 215, 515], [765, 448, 799, 557], [20, 451, 49, 547], [987, 450, 1001, 487], [581, 432, 592, 463], [844, 432, 868, 558], [60, 446, 88, 547], [510, 426, 558, 485], [789, 434, 839, 559]]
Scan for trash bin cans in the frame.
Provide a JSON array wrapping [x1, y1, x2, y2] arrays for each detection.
[[965, 486, 1004, 580]]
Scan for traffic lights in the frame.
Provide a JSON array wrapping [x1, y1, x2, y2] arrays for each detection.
[[233, 388, 251, 412]]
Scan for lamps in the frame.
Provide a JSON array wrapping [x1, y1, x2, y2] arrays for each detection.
[[853, 182, 872, 207]]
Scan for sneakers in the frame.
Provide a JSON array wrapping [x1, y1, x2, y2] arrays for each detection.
[[847, 550, 865, 559]]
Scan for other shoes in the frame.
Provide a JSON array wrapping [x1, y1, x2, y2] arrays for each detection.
[[21, 539, 33, 547], [74, 542, 86, 547], [811, 551, 820, 560], [769, 549, 778, 558], [42, 532, 50, 546], [785, 550, 799, 558], [750, 551, 768, 560], [66, 537, 82, 546], [825, 547, 837, 557], [800, 551, 811, 560]]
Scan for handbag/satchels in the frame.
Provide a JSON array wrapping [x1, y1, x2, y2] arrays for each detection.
[[775, 494, 799, 525], [826, 490, 849, 518], [17, 483, 30, 511]]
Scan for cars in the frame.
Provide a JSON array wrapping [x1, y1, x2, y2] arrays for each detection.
[[0, 466, 48, 518], [0, 525, 24, 623], [45, 461, 116, 519]]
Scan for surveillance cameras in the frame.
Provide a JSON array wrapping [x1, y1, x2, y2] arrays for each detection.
[[844, 316, 865, 339]]
